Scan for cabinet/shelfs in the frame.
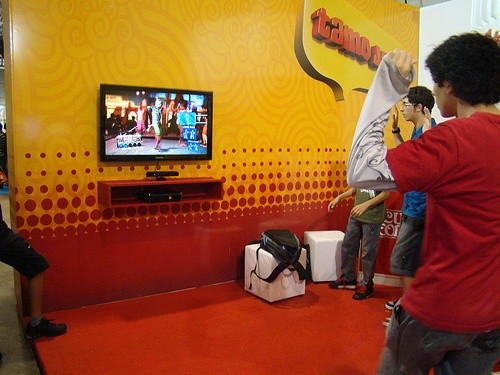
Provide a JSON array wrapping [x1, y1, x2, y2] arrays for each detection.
[[97, 177, 224, 209]]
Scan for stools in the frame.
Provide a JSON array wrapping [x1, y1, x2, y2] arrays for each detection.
[[303, 230, 346, 282], [244, 241, 307, 303]]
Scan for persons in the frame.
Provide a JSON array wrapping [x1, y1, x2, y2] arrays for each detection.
[[328, 86, 440, 301], [0, 122, 67, 360], [135, 97, 208, 149], [347, 28, 500, 375]]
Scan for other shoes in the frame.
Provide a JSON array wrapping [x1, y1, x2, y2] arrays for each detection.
[[385, 300, 398, 309], [382, 317, 391, 326]]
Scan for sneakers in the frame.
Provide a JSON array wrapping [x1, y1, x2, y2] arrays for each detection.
[[25, 317, 67, 339], [352, 284, 374, 300], [329, 274, 356, 288]]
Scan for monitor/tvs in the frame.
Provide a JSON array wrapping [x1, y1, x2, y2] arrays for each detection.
[[99, 83, 214, 161]]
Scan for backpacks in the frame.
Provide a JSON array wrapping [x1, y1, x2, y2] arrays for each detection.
[[253, 229, 311, 283]]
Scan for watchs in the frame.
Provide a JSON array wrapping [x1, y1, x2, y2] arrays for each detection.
[[391, 128, 400, 133]]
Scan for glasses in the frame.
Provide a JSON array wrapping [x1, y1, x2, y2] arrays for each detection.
[[402, 101, 416, 107]]
